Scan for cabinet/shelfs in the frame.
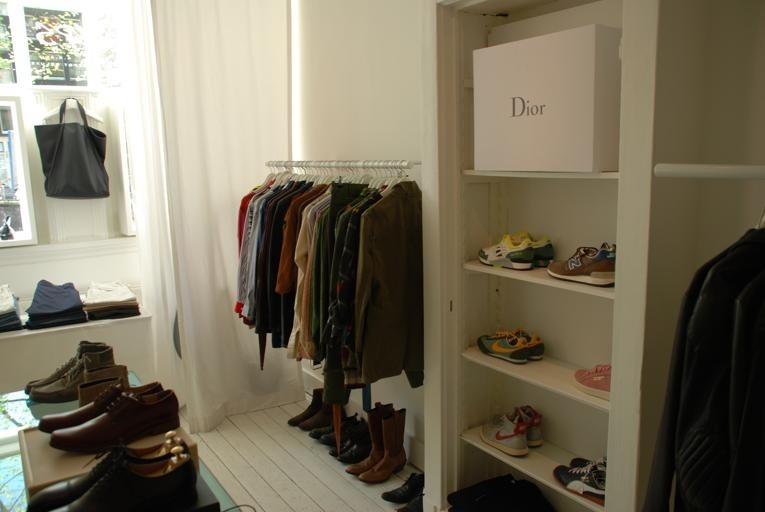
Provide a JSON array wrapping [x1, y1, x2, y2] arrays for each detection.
[[460, 169, 619, 512]]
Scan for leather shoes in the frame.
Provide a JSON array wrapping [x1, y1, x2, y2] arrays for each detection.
[[382, 472, 425, 511], [310, 412, 372, 463], [25, 381, 199, 512]]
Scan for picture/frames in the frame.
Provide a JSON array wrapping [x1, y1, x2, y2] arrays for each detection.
[[0, 96, 38, 248]]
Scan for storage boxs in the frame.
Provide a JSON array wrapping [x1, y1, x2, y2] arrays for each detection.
[[187, 468, 221, 512], [473, 25, 622, 173]]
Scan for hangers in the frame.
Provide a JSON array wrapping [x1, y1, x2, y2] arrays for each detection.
[[263, 161, 413, 197]]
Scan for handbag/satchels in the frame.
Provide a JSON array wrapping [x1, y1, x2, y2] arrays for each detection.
[[35, 97, 110, 199]]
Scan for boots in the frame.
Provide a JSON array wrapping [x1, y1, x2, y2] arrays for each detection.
[[25, 340, 129, 407], [345, 402, 393, 474], [359, 408, 407, 483], [288, 388, 346, 429]]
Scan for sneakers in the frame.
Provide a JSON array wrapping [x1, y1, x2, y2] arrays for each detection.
[[477, 232, 617, 508]]
